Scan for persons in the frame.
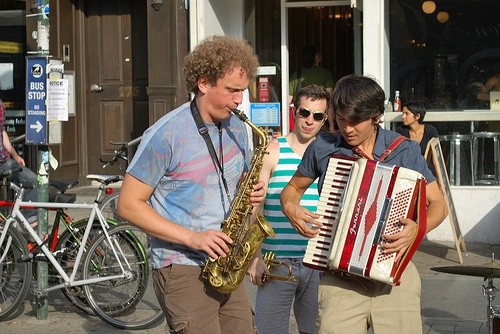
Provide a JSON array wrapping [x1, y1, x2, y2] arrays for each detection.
[[0, 98, 38, 201], [395, 100, 438, 157], [246, 84, 331, 334], [280, 74, 448, 334], [289, 45, 335, 132], [464, 73, 500, 175], [117, 34, 266, 334]]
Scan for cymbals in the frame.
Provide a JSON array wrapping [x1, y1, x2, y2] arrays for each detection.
[[429, 262, 500, 279]]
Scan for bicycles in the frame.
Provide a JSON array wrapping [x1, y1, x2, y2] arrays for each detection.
[[0, 136, 166, 330]]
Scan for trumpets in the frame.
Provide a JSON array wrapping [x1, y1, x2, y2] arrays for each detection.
[[243, 251, 297, 285]]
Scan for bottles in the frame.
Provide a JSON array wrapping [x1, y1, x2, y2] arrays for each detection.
[[26, 240, 38, 253], [388, 98, 392, 112], [394, 91, 401, 112]]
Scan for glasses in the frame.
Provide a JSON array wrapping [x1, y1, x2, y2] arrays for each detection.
[[299, 106, 324, 121]]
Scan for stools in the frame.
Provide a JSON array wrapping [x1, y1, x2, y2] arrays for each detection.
[[470, 132, 500, 187], [439, 135, 472, 187]]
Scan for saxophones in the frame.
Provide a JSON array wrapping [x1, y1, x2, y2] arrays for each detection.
[[201, 108, 276, 294]]
